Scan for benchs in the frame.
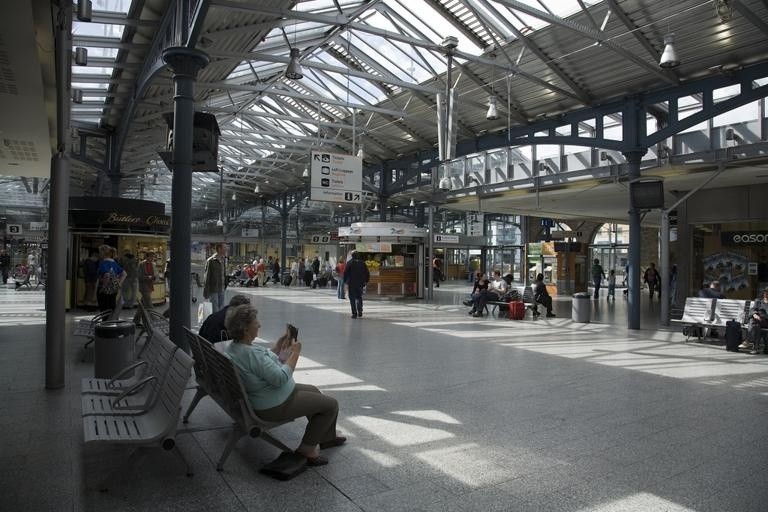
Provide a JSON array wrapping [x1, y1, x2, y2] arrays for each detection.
[[134, 300, 170, 358], [80, 326, 195, 492], [182, 324, 296, 472], [74, 295, 127, 347], [35, 271, 46, 289], [670, 296, 767, 349], [483, 287, 539, 319], [16, 272, 32, 291]]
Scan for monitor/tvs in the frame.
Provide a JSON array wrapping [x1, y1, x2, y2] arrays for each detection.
[[629, 181, 666, 208]]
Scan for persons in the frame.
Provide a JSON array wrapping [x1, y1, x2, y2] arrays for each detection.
[[226, 254, 279, 287], [78, 245, 158, 329], [202, 241, 229, 311], [223, 304, 347, 465], [589, 258, 605, 300], [643, 264, 661, 297], [463, 269, 514, 317], [736, 287, 768, 356], [696, 279, 723, 299], [197, 293, 251, 342], [433, 255, 442, 289], [343, 249, 370, 318], [335, 258, 346, 300], [605, 268, 618, 300], [530, 271, 557, 317], [289, 255, 333, 288], [1, 247, 42, 288]]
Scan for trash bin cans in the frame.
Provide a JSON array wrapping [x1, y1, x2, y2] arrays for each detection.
[[95, 320, 136, 380], [282, 272, 290, 286], [572, 292, 590, 322]]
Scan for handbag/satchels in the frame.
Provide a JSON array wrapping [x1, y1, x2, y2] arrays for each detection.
[[198, 301, 212, 324], [101, 277, 121, 294]]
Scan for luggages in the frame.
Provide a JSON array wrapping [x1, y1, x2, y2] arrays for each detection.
[[284, 276, 292, 286], [725, 319, 741, 352], [509, 301, 525, 320]]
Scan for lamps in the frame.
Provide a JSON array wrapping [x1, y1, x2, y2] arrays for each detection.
[[486, 65, 502, 124], [254, 183, 260, 194], [658, 19, 680, 69], [232, 192, 236, 200], [302, 164, 310, 178], [285, 3, 303, 80], [356, 137, 366, 160]]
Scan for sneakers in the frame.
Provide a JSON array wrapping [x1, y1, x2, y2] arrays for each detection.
[[351, 309, 363, 318], [322, 436, 346, 446], [533, 312, 540, 316], [463, 300, 483, 317], [738, 342, 760, 355], [546, 312, 556, 317], [295, 448, 329, 465]]
